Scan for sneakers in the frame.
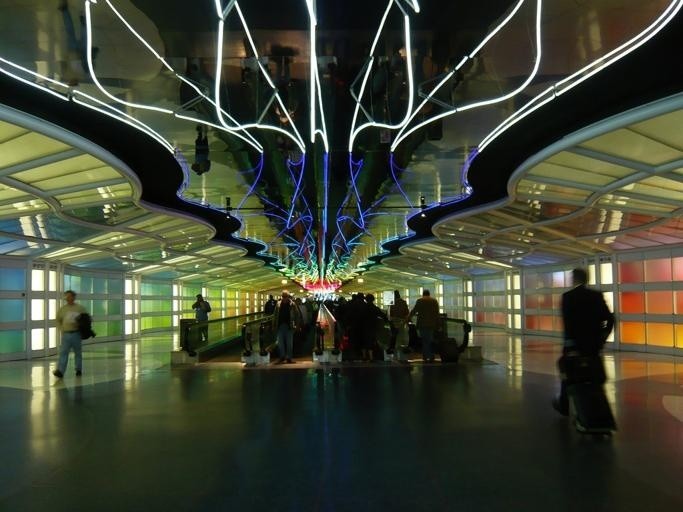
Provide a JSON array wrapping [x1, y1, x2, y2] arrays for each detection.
[[279, 358, 296, 363], [76, 370, 81, 376], [53, 369, 63, 377], [552, 396, 570, 416]]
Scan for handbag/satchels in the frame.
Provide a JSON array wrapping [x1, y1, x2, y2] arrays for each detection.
[[558, 354, 606, 392]]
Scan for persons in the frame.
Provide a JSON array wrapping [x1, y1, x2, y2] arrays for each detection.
[[57, 1, 92, 89], [50, 290, 88, 379], [191, 293, 210, 342], [175, 38, 455, 174], [261, 287, 443, 366], [551, 267, 615, 420]]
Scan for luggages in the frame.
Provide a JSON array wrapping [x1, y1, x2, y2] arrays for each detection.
[[568, 387, 617, 437]]
